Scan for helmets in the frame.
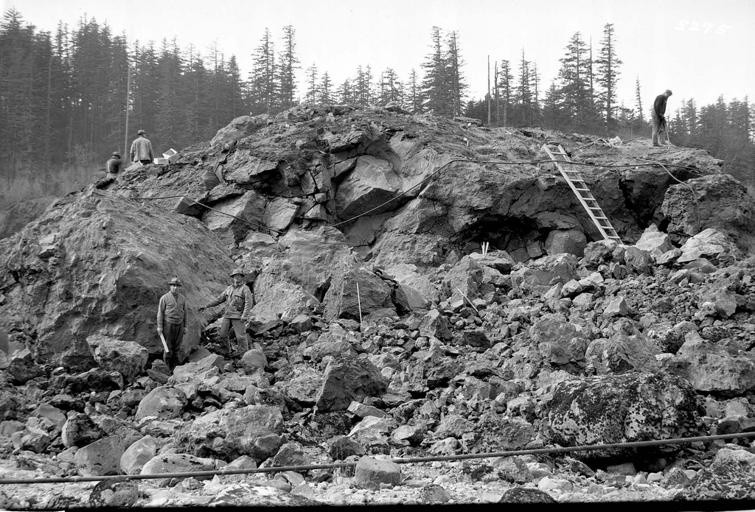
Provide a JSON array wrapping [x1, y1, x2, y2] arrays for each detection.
[[138, 130, 146, 134], [230, 269, 246, 277], [112, 152, 121, 158], [168, 279, 183, 285]]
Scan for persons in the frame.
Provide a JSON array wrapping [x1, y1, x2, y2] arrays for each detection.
[[648, 89, 672, 148], [104, 152, 121, 179], [197, 267, 253, 357], [157, 276, 188, 375], [129, 128, 153, 166]]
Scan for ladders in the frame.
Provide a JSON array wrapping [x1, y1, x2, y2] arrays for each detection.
[[543, 143, 624, 245]]
